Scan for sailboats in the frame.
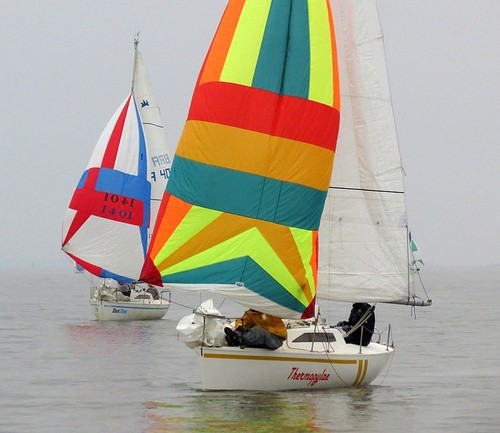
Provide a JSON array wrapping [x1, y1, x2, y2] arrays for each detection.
[[59, 30, 175, 321], [129, 0, 434, 393]]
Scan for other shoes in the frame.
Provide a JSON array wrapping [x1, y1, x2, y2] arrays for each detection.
[[222, 325, 237, 345]]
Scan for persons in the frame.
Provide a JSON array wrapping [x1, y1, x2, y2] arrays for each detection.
[[113, 281, 129, 296], [338, 302, 375, 346], [225, 307, 287, 350], [145, 284, 159, 299]]
